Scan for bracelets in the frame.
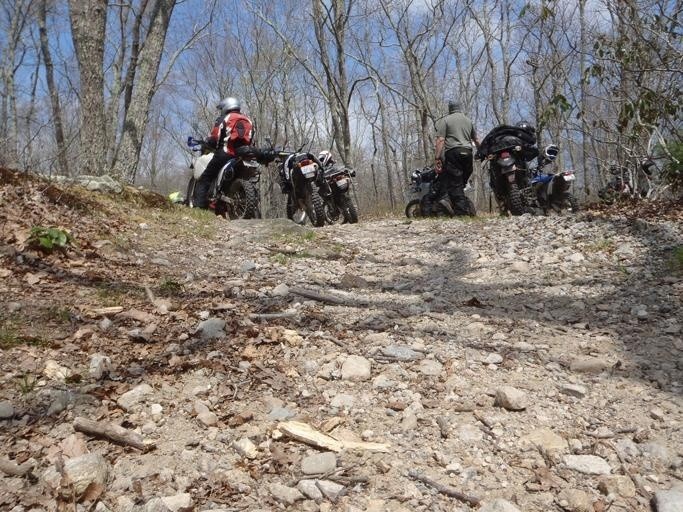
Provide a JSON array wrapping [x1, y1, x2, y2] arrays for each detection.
[[434, 157, 441, 160]]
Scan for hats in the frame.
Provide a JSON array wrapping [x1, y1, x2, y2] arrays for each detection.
[[448, 99, 461, 111]]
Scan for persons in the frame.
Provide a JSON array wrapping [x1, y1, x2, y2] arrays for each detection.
[[193, 97, 260, 219], [433, 100, 480, 218]]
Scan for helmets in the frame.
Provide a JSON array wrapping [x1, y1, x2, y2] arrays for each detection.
[[515, 120, 535, 131], [215, 96, 240, 112], [318, 149, 332, 166]]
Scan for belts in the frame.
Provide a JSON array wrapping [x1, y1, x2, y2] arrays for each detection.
[[454, 145, 472, 150]]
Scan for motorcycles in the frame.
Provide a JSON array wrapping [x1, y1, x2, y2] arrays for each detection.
[[598, 167, 647, 205], [526, 146, 579, 212], [405, 163, 476, 216], [262, 134, 359, 227], [474, 135, 533, 215], [186, 136, 257, 220]]
